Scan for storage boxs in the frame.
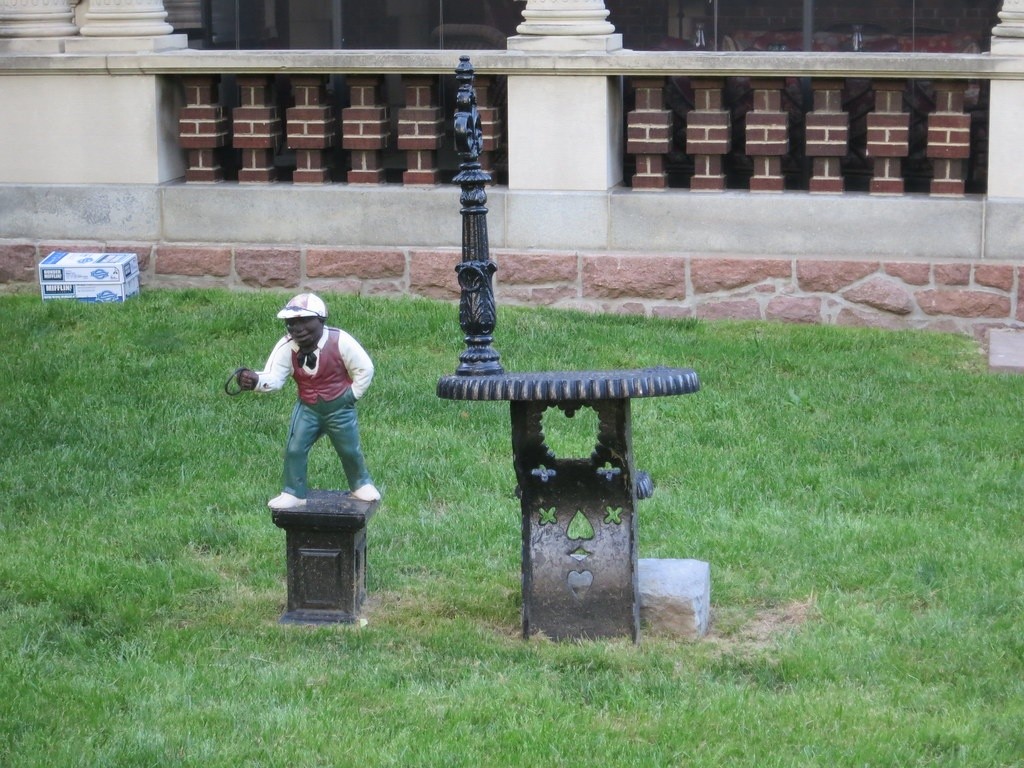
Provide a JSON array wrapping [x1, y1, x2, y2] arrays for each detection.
[[38, 251, 140, 303]]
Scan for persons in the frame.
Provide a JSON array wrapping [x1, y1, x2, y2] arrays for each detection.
[[236, 292, 382, 509]]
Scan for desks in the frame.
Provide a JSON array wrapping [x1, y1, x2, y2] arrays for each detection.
[[434, 368, 699, 645]]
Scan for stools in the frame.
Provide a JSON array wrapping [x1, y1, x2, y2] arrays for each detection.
[[269, 492, 380, 624]]
